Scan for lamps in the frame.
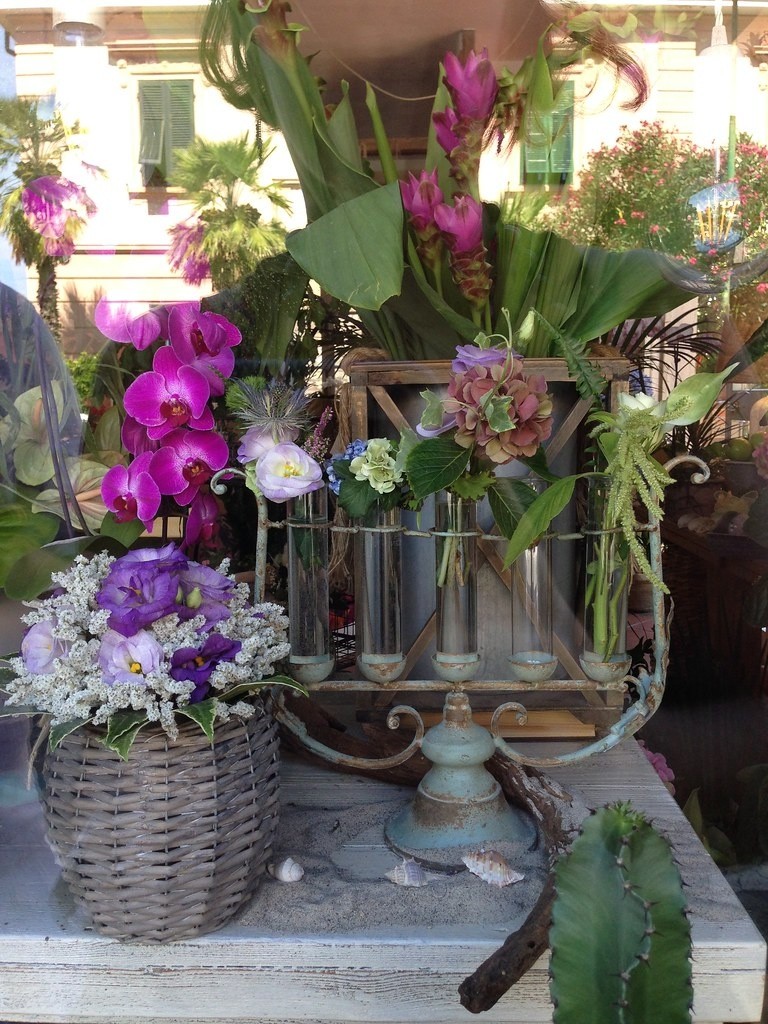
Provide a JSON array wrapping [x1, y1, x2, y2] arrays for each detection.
[[50, 0, 108, 41]]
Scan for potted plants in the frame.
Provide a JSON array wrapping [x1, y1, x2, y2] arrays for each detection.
[[653, 340, 759, 485]]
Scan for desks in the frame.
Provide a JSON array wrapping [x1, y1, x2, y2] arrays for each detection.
[[633, 485, 768, 696], [1, 723, 768, 1022]]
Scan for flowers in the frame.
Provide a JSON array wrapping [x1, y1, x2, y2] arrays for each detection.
[[3, 5, 765, 542], [1, 544, 311, 758]]
